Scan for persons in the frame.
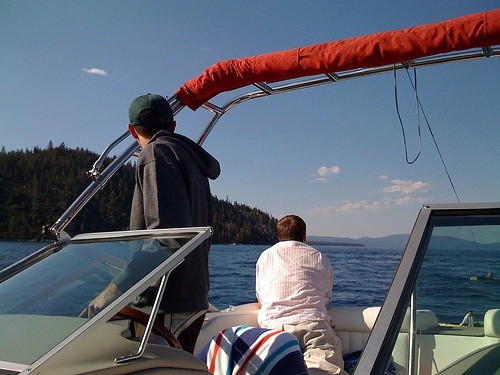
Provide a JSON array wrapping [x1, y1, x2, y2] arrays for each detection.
[[487, 271, 495, 278], [85, 93, 221, 358], [255, 215, 349, 375]]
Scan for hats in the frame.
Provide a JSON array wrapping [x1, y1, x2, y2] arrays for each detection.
[[129, 93, 173, 126]]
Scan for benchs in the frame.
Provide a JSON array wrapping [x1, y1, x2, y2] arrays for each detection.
[[193, 300, 437, 360]]
